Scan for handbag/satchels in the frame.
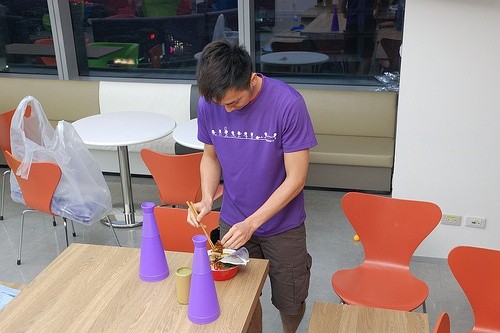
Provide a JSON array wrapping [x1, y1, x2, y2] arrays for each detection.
[[212, 14, 239, 48], [10, 95, 113, 226]]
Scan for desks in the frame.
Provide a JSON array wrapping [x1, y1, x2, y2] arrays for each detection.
[[261, 5, 404, 73], [69, 112, 177, 228], [4, 44, 124, 59], [172, 117, 206, 153], [305, 301, 430, 333]]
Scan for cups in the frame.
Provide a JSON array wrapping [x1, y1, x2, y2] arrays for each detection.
[[176, 266, 192, 305]]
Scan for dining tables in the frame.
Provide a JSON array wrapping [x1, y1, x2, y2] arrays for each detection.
[[0, 243, 270, 333]]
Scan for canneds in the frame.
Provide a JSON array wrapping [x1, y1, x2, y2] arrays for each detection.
[[293, 16, 298, 26]]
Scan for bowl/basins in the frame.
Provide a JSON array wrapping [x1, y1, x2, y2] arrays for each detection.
[[206, 248, 239, 280]]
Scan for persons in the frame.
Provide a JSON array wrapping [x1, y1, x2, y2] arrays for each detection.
[[340, 0, 404, 73], [187, 40, 318, 333], [0, 0, 192, 67]]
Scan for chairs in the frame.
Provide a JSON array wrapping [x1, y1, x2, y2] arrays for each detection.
[[0, 104, 500, 333]]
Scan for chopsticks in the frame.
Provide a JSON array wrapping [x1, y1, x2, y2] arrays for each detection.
[[186, 200, 215, 249]]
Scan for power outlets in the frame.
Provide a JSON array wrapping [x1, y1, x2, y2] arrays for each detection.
[[441, 215, 461, 225], [465, 216, 487, 229]]
